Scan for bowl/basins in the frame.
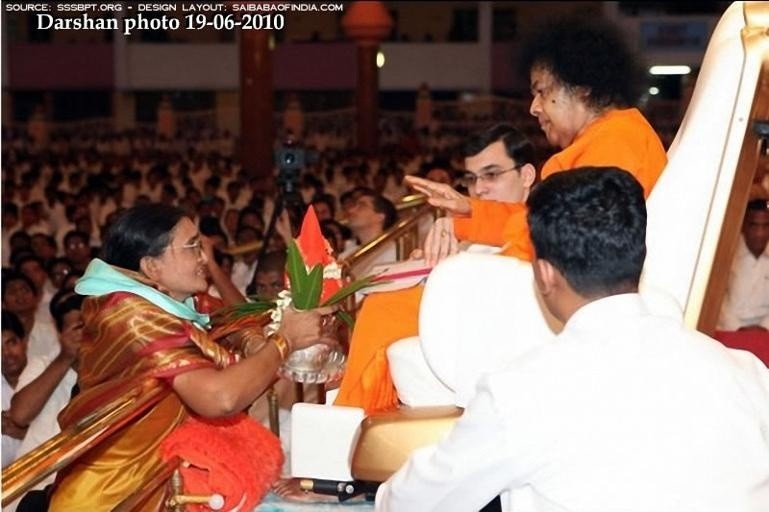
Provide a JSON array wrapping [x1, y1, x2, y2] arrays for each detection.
[[280, 349, 345, 384]]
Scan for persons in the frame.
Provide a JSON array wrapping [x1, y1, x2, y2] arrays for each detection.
[[331, 1, 674, 415], [713, 135, 769, 342], [372, 164, 769, 512], [1, 106, 559, 512]]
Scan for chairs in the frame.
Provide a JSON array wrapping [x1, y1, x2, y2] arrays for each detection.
[[290, 0, 768, 502]]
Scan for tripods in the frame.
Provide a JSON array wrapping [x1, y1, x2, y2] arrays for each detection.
[[249, 171, 307, 302]]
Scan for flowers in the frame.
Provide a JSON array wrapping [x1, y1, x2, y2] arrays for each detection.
[[205, 203, 394, 383]]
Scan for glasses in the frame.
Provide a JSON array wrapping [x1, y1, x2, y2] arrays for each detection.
[[158, 237, 203, 259], [461, 163, 525, 188]]
